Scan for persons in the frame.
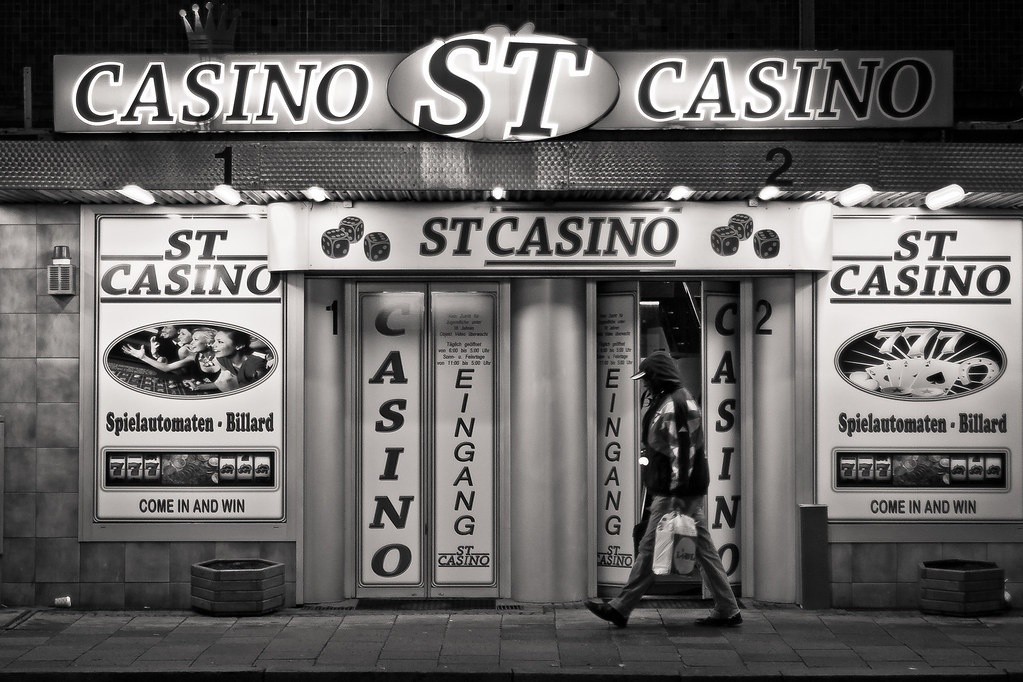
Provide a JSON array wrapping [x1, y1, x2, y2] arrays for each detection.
[[584, 352, 744, 630], [122, 325, 271, 394]]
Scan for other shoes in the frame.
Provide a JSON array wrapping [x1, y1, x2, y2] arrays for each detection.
[[584, 600, 627, 628], [695, 611, 742, 627]]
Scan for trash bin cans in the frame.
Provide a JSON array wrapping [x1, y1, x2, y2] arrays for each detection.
[[795, 502, 833, 609]]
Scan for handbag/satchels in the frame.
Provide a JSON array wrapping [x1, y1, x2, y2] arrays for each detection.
[[652, 507, 698, 576]]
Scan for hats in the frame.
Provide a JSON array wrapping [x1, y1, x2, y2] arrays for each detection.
[[631, 371, 647, 380]]
